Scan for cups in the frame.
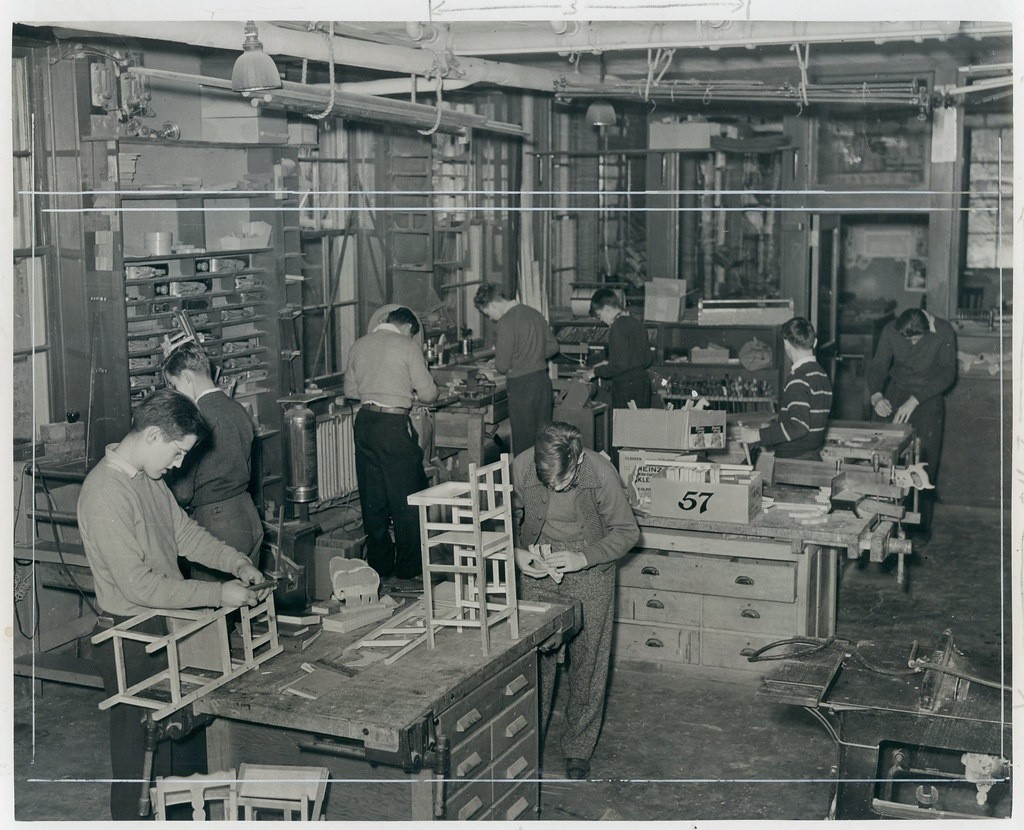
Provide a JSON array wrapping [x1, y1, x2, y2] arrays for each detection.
[[459, 340, 472, 356], [436, 350, 450, 365]]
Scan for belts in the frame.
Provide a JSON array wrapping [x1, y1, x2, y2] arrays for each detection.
[[361, 403, 410, 415]]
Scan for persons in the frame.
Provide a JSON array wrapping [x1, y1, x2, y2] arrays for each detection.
[[76, 389, 277, 821], [867, 308, 957, 548], [732, 317, 833, 460], [583, 288, 653, 467], [160, 341, 264, 583], [344, 307, 447, 584], [494, 423, 641, 779], [473, 282, 560, 456]]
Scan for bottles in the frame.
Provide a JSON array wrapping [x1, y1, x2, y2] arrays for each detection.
[[425, 338, 433, 359]]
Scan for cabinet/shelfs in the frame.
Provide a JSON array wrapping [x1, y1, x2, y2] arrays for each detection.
[[121, 246, 272, 411], [437, 648, 543, 820], [609, 547, 809, 686]]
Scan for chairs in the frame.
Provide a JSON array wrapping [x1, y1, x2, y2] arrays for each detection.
[[145, 763, 329, 822], [91, 581, 283, 723]]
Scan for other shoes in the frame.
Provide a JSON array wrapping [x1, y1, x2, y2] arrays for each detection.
[[565, 758, 591, 779], [911, 537, 928, 551], [396, 571, 448, 583]]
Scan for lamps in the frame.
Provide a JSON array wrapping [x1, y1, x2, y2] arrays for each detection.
[[231, 21, 281, 92], [585, 51, 618, 126]]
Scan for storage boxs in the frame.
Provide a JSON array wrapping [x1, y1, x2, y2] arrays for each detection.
[[612, 408, 764, 525]]
